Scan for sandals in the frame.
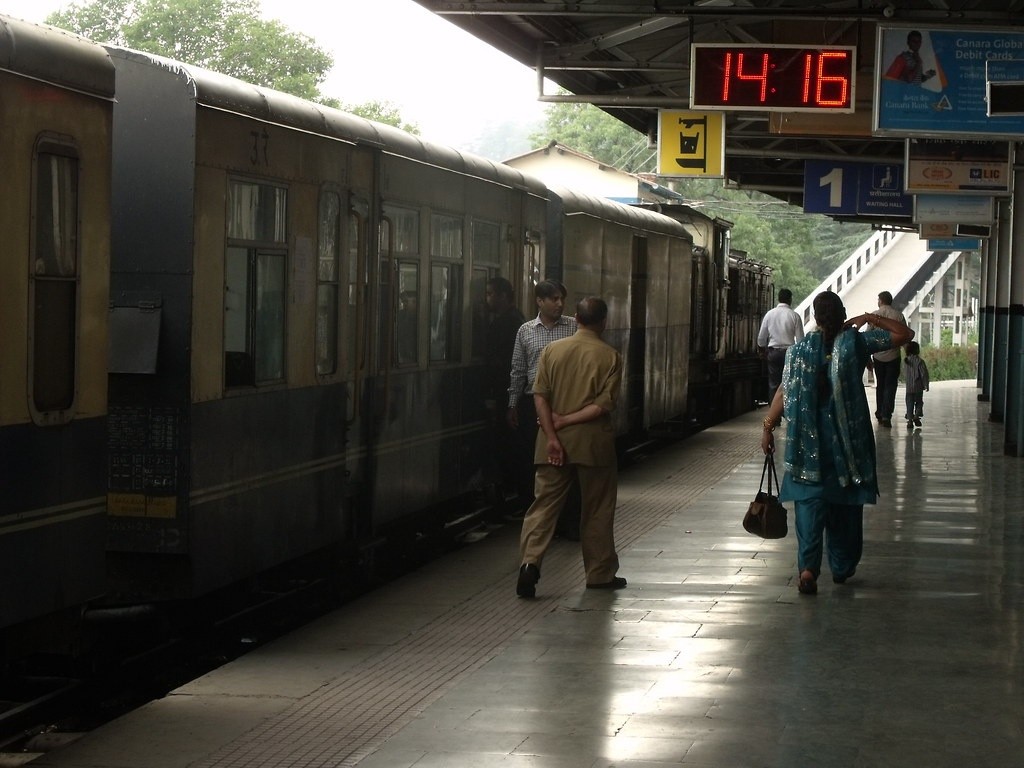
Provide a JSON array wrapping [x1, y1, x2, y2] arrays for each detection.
[[797, 567, 818, 594], [833, 567, 856, 584]]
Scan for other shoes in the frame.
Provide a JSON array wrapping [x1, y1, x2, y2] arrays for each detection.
[[913, 417, 923, 426], [555, 519, 581, 542], [907, 421, 914, 428], [877, 415, 892, 428], [504, 507, 526, 522]]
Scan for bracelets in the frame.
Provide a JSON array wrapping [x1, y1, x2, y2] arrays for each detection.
[[763, 415, 775, 432], [864, 312, 882, 327]]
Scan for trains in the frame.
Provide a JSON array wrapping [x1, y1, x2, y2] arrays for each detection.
[[1, 16, 780, 702]]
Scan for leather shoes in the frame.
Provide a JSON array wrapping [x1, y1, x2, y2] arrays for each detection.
[[585, 574, 627, 589], [516, 562, 540, 598]]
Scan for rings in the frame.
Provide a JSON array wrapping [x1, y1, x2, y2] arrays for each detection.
[[764, 451, 766, 455]]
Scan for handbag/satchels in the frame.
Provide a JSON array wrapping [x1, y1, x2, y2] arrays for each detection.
[[742, 447, 789, 540]]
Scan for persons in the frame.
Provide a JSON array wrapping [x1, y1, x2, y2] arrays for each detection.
[[865, 291, 910, 427], [761, 291, 915, 594], [756, 288, 805, 426], [515, 296, 627, 598], [485, 277, 537, 522], [505, 277, 581, 540], [906, 341, 929, 429], [884, 28, 937, 91]]
